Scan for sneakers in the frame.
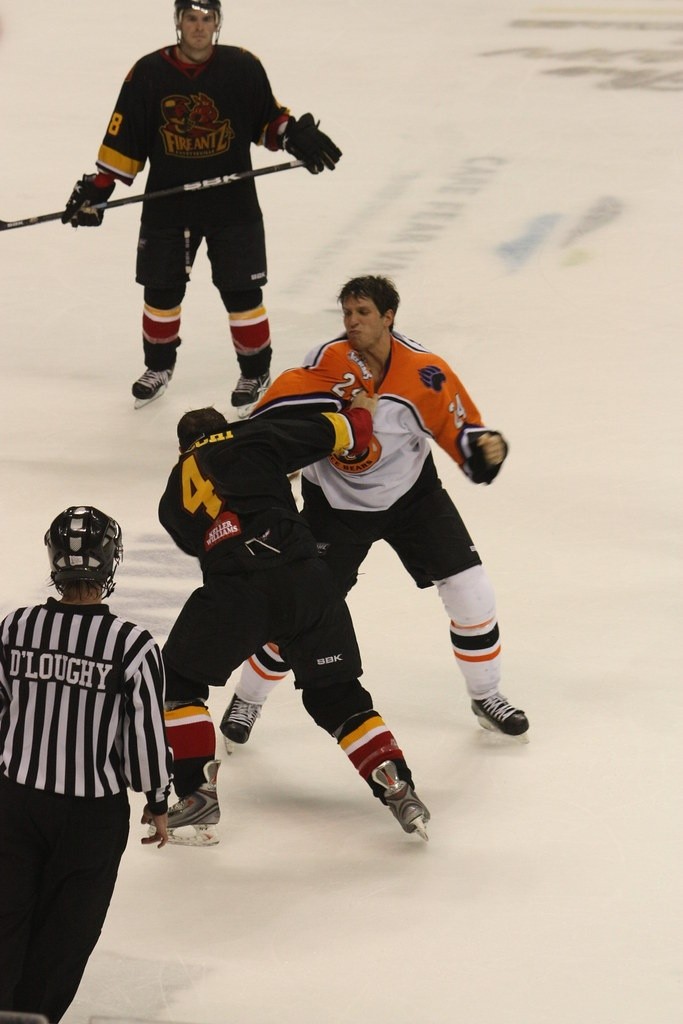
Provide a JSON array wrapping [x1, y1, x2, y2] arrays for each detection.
[[231, 373, 272, 418], [147, 759, 220, 846], [473, 692, 530, 745], [219, 693, 262, 754], [132, 368, 173, 410], [370, 759, 432, 841]]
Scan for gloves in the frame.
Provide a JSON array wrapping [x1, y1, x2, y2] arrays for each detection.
[[62, 174, 115, 228], [286, 112, 342, 175]]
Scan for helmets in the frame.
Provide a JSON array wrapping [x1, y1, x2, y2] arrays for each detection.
[[175, 0, 222, 29], [44, 506, 121, 583]]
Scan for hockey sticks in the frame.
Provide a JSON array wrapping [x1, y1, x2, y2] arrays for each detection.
[[0, 158, 306, 232]]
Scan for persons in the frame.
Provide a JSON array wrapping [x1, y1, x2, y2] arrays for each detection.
[[152, 408, 431, 841], [63, 0, 343, 419], [220, 275, 531, 756], [0, 507, 177, 1024]]
